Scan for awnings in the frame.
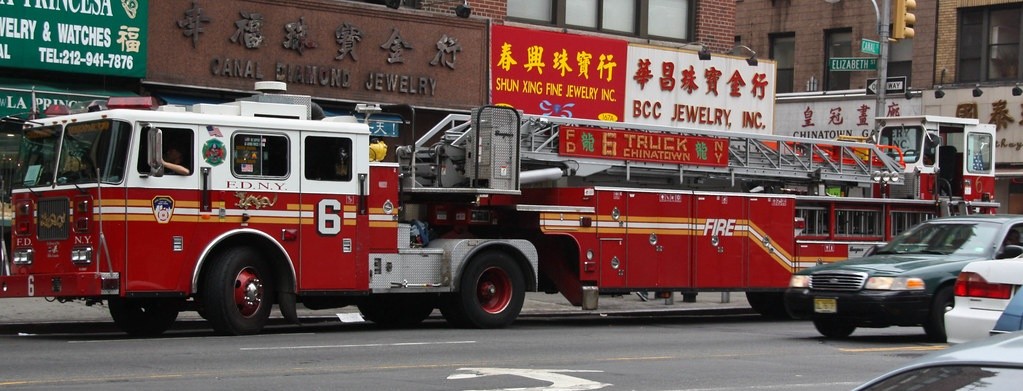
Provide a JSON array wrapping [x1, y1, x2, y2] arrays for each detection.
[[0, 80, 142, 126], [158, 92, 403, 138]]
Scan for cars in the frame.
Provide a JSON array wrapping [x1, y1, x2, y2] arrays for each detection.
[[942, 251, 1023, 347], [781, 214, 1023, 340], [849, 330, 1023, 391]]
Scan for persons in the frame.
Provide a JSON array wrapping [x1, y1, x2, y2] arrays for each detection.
[[996, 229, 1023, 259], [161, 143, 192, 175]]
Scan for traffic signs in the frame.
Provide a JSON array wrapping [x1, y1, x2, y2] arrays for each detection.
[[865, 75, 907, 96]]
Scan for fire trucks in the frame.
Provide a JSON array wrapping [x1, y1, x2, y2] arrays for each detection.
[[0, 80, 999, 335]]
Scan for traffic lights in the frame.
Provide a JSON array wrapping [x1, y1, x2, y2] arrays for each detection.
[[891, 0, 916, 41]]
